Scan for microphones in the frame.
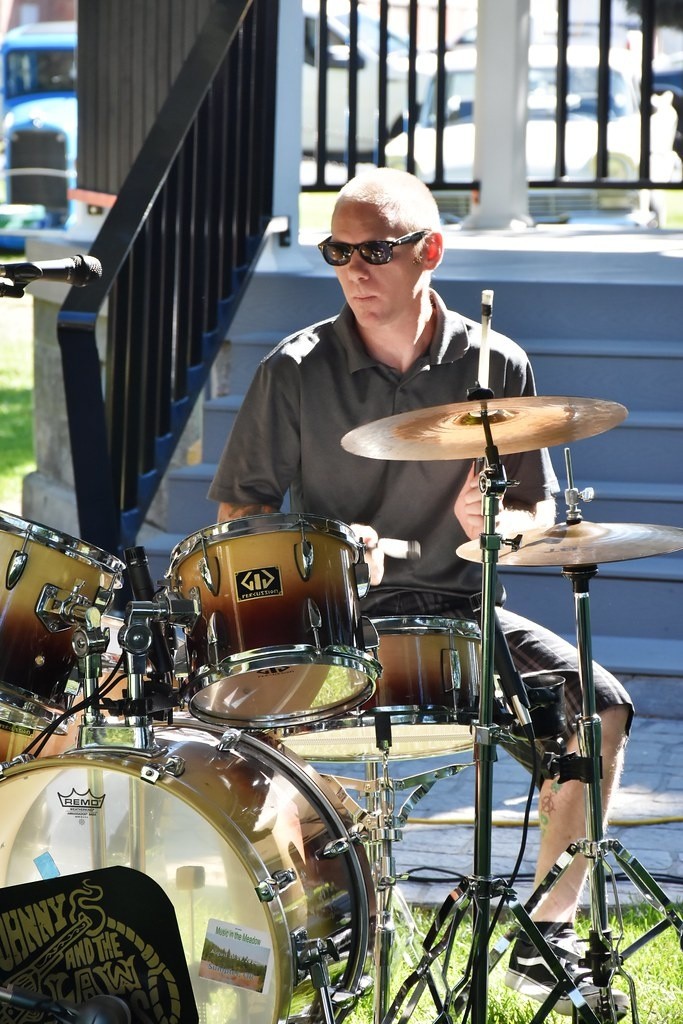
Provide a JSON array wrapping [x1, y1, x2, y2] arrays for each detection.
[[124, 545, 175, 687], [0, 254, 103, 287], [469, 593, 536, 744]]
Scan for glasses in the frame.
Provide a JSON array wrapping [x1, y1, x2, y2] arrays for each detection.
[[318, 230, 431, 267]]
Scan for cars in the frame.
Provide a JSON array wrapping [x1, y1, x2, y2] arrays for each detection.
[[301, 5, 439, 160], [382, 47, 677, 190], [457, 15, 682, 155]]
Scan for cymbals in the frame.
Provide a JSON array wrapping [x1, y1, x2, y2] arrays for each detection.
[[454, 521, 683, 568], [340, 396, 629, 461]]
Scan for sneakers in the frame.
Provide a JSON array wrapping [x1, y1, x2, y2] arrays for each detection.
[[504, 929, 630, 1016]]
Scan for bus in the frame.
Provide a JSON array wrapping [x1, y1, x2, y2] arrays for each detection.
[[0, 21, 78, 118]]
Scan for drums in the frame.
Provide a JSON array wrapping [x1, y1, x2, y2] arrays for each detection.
[[0, 509, 128, 738], [164, 514, 384, 730], [0, 720, 378, 1024], [263, 616, 483, 765], [0, 613, 174, 774]]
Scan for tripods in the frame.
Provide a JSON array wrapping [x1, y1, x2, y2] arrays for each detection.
[[361, 412, 683, 1024]]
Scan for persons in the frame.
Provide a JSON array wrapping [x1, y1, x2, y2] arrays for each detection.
[[207, 167, 637, 1015]]
